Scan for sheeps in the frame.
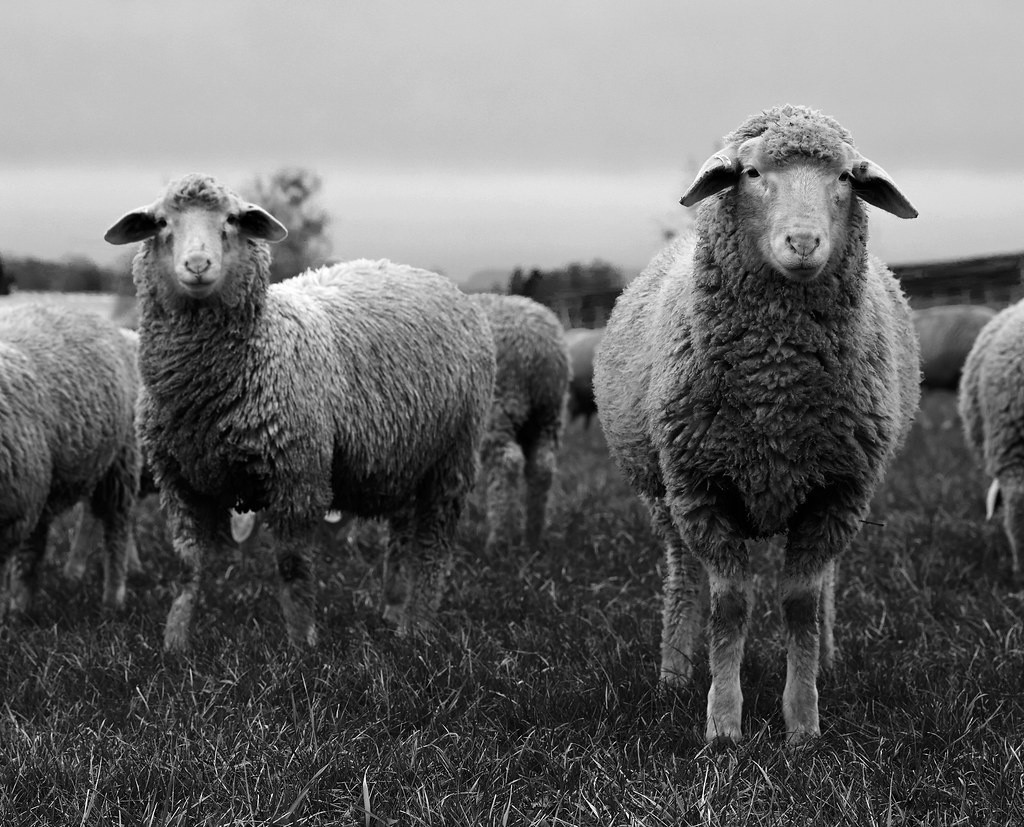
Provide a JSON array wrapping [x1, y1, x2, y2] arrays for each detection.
[[463, 291, 574, 550], [0, 306, 159, 611], [104, 174, 500, 661], [955, 295, 1022, 577], [589, 103, 926, 739]]
